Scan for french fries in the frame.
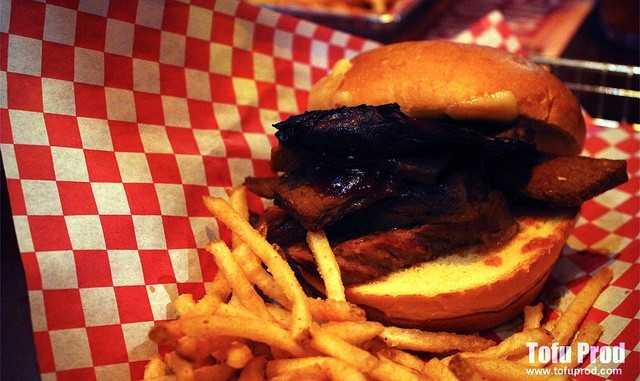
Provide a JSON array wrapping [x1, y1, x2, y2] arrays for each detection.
[[140, 184, 613, 380]]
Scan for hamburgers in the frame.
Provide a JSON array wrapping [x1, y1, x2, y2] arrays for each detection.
[[242, 39, 630, 336]]
